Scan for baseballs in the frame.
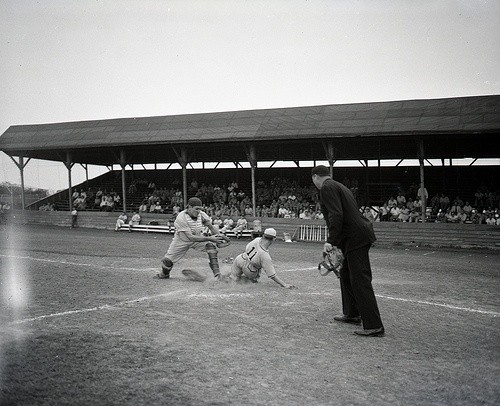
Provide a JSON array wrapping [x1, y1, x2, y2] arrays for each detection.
[[222, 240, 228, 244]]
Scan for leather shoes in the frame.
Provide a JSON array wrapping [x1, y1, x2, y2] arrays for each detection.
[[333, 315, 361, 326], [354, 327, 385, 336]]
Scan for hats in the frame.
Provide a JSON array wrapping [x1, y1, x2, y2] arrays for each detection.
[[263, 228, 276, 239], [185, 197, 202, 209]]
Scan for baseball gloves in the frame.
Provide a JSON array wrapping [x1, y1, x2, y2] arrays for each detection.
[[216, 234, 231, 249]]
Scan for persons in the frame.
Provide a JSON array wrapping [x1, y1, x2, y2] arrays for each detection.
[[152, 197, 229, 280], [39, 202, 59, 211], [363, 183, 500, 225], [440, 192, 449, 210], [0, 202, 10, 210], [71, 208, 77, 227], [72, 187, 120, 212], [114, 212, 128, 231], [208, 216, 222, 235], [311, 166, 384, 337], [390, 203, 400, 222], [234, 215, 248, 239], [129, 176, 358, 212], [222, 227, 292, 288], [251, 218, 261, 239], [397, 192, 406, 207], [129, 212, 140, 233], [204, 217, 212, 236], [168, 211, 179, 233], [222, 215, 233, 235]]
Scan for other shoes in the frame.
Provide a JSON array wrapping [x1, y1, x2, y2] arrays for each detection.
[[154, 273, 169, 279]]
